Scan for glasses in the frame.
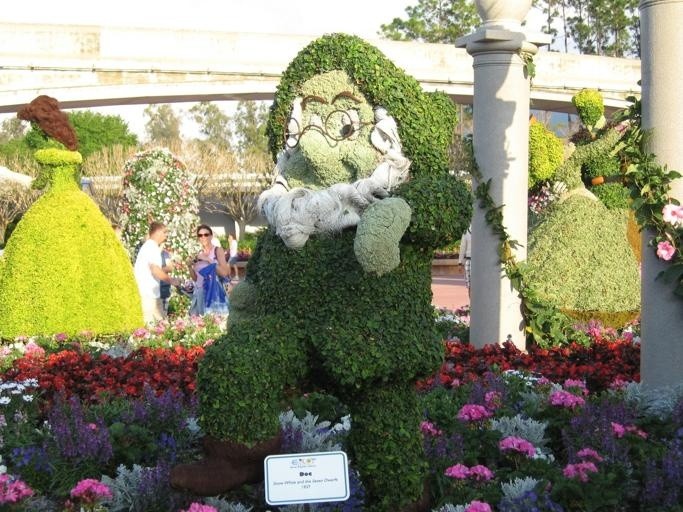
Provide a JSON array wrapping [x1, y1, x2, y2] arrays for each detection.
[[196, 233, 210, 237]]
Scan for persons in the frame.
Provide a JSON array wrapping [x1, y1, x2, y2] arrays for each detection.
[[458, 221, 472, 304], [224, 234, 239, 283], [185, 224, 229, 318], [134, 221, 181, 333], [158, 245, 174, 317]]
